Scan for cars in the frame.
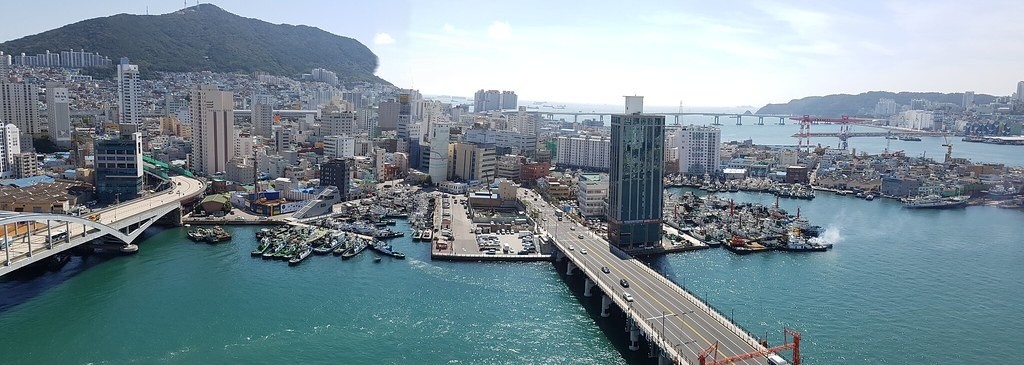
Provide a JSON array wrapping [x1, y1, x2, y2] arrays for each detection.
[[474, 226, 535, 255], [568, 245, 574, 250], [558, 216, 562, 222], [542, 217, 547, 221], [580, 249, 587, 254], [619, 279, 629, 288], [578, 234, 584, 240], [601, 266, 610, 274], [624, 292, 633, 302], [570, 226, 575, 231]]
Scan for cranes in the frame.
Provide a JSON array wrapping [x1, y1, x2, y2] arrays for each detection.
[[791, 130, 950, 150], [789, 114, 888, 131]]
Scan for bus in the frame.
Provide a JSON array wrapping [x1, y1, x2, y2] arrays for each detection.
[[555, 210, 563, 217]]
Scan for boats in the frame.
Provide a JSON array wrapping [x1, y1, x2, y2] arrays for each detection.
[[664, 188, 834, 254], [317, 190, 433, 258], [666, 174, 818, 199], [186, 224, 231, 244], [251, 227, 369, 266], [835, 189, 875, 201], [899, 193, 971, 209]]
